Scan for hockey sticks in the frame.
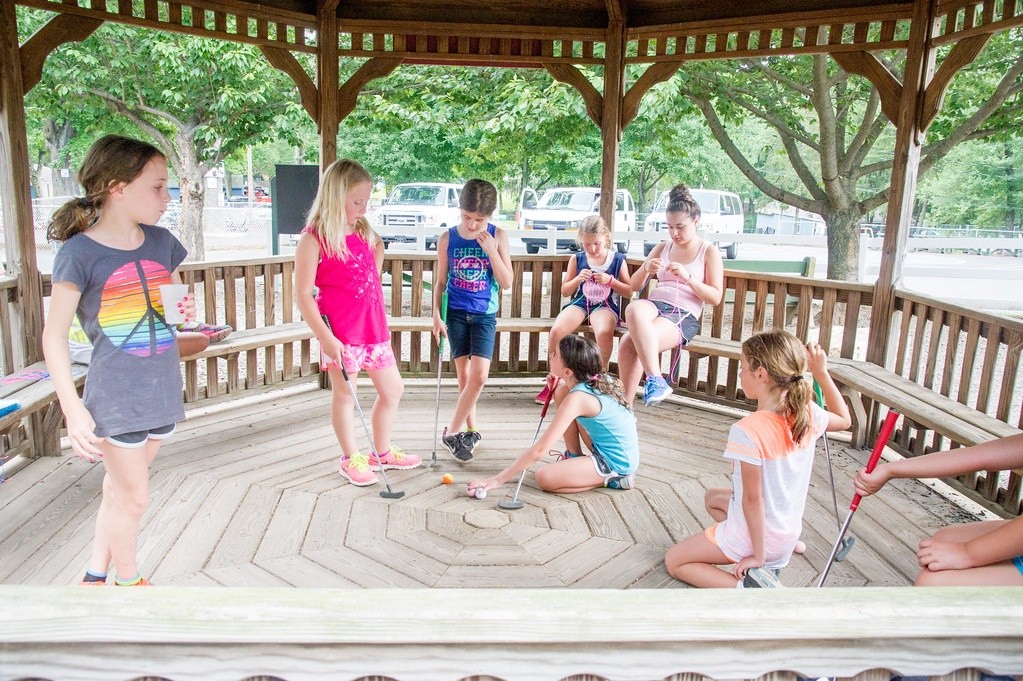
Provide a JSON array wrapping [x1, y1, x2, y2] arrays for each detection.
[[429, 290, 450, 468], [320, 313, 406, 498], [498, 376, 558, 509], [812, 403, 901, 585], [813, 380, 856, 560]]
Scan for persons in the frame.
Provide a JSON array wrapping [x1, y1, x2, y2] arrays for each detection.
[[41, 134, 233, 586], [664, 330, 851, 588], [534, 215, 633, 405], [853, 432, 1023, 586], [618, 184, 723, 423], [433, 178, 514, 463], [467, 334, 640, 494], [294, 158, 422, 487]]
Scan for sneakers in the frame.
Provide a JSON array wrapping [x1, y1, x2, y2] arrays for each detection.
[[178, 322, 233, 341], [548, 449, 585, 462], [367, 448, 422, 471], [644, 373, 673, 407], [339, 452, 378, 487], [441, 426, 474, 464], [458, 431, 481, 452], [610, 475, 634, 490], [535, 385, 554, 404], [742, 567, 782, 587]]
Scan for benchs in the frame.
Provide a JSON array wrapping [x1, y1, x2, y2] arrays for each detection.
[[720, 257, 816, 328], [372, 251, 588, 379], [1, 281, 88, 462], [828, 283, 1023, 512], [616, 255, 874, 452], [172, 255, 327, 410]]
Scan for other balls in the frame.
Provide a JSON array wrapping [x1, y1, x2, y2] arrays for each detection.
[[466, 487, 476, 497], [442, 473, 454, 484], [796, 541, 806, 553], [475, 488, 487, 499]]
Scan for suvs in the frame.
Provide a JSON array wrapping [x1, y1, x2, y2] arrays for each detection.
[[520, 186, 637, 254], [643, 188, 745, 259], [372, 182, 467, 249]]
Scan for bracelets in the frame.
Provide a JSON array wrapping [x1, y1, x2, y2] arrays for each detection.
[[607, 275, 614, 286]]
